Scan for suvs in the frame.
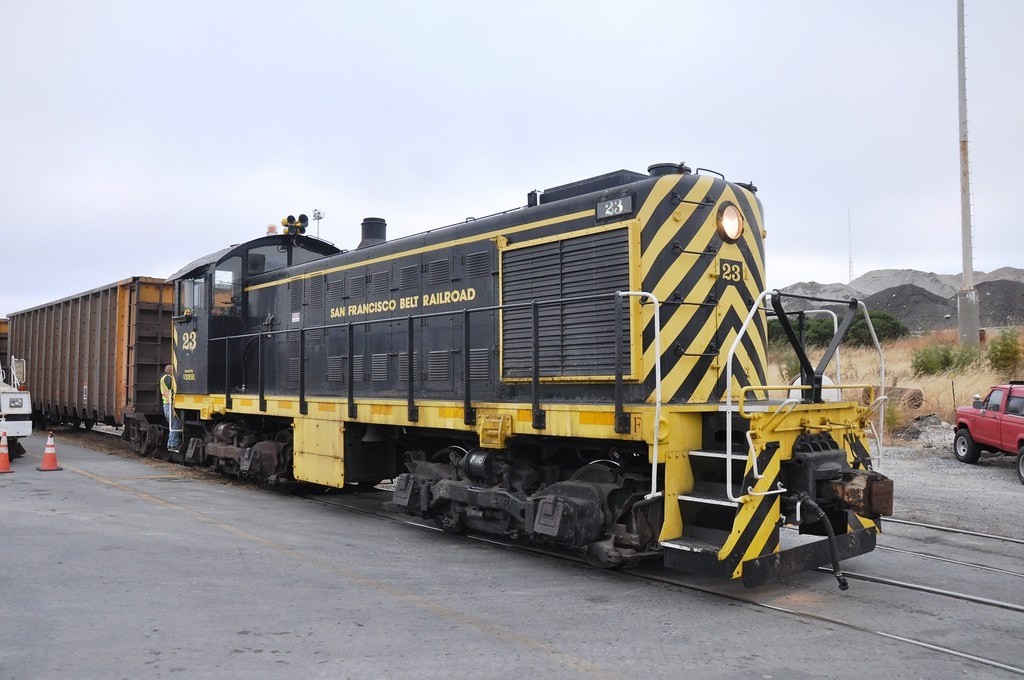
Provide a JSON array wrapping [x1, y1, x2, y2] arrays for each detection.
[[953, 379, 1024, 485]]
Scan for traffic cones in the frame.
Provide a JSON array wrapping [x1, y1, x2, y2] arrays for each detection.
[[34, 432, 63, 472], [0, 432, 15, 473]]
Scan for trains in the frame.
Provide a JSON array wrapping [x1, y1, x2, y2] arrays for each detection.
[[0, 161, 894, 591]]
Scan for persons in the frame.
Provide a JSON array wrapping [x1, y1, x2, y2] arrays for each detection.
[[160, 365, 181, 453]]
